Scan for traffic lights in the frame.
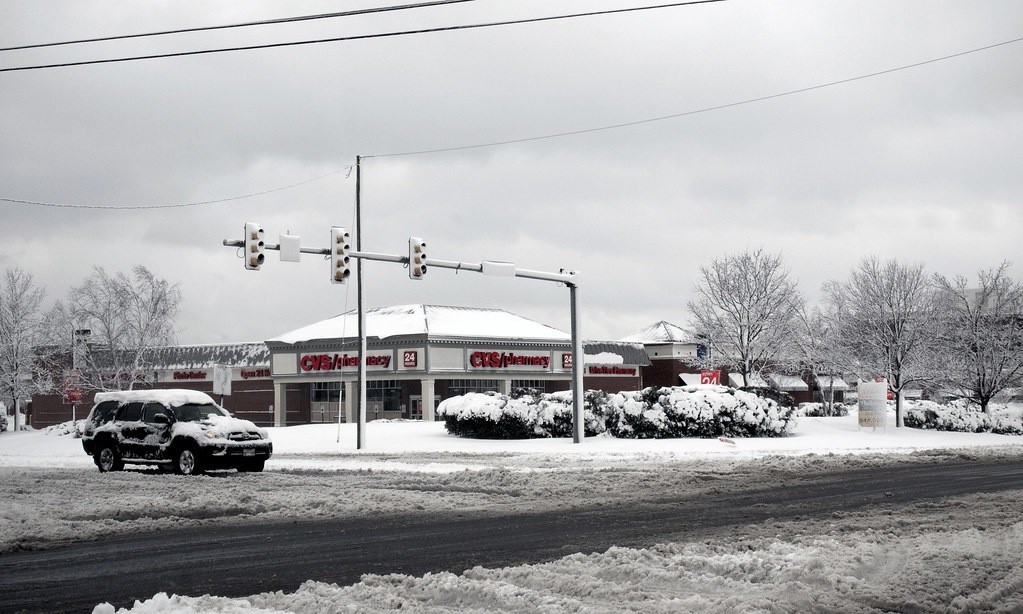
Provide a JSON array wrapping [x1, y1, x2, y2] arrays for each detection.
[[409, 236, 427, 280], [245, 222, 265, 270], [331, 228, 351, 284]]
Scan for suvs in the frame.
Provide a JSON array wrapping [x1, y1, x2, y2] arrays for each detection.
[[79, 390, 275, 475]]
